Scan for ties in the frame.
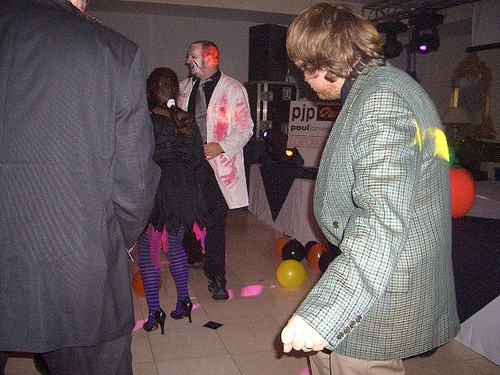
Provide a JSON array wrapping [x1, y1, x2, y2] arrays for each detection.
[[195, 78, 213, 143]]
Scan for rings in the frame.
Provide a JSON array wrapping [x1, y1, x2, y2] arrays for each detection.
[[205, 155, 210, 159]]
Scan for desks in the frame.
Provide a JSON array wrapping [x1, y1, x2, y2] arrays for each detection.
[[245, 155, 500, 366]]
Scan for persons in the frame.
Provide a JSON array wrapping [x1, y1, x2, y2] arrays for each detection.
[[280, 3, 461, 375], [175, 41, 255, 300], [138, 67, 215, 335], [0, 0, 162, 375]]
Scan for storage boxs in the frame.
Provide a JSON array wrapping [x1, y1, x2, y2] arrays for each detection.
[[243, 81, 299, 141]]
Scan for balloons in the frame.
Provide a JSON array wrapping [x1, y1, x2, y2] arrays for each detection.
[[276, 259, 309, 288], [305, 241, 318, 260], [309, 243, 329, 272], [282, 238, 307, 262], [450, 168, 475, 219], [275, 235, 290, 257], [319, 244, 341, 273]]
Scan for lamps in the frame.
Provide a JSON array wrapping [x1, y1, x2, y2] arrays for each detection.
[[376, 7, 445, 59]]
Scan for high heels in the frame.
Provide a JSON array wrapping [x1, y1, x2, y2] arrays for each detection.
[[143, 308, 166, 334], [171, 298, 192, 323]]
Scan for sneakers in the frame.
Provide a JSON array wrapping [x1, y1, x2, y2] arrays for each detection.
[[187, 255, 206, 267], [208, 274, 229, 299]]
